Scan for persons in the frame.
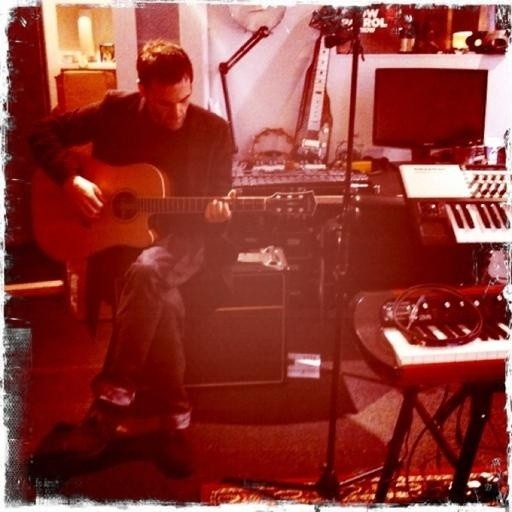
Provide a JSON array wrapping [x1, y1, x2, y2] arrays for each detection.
[[28, 39, 237, 474]]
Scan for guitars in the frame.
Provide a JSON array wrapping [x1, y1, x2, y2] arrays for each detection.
[[31, 151, 319, 258]]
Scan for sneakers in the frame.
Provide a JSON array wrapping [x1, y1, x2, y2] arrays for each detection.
[[162, 431, 193, 475], [64, 401, 119, 454]]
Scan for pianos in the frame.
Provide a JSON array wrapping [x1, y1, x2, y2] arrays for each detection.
[[396, 161, 512, 249], [347, 283, 508, 390]]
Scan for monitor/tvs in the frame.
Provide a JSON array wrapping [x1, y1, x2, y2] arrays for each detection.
[[370, 68, 489, 167]]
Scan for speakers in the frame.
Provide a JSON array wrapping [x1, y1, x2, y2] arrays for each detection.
[[183, 272, 289, 390]]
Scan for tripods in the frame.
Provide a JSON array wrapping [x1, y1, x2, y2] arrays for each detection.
[[224, 42, 385, 502]]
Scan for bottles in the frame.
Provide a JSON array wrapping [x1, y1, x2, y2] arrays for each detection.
[[399, 14, 415, 52]]
